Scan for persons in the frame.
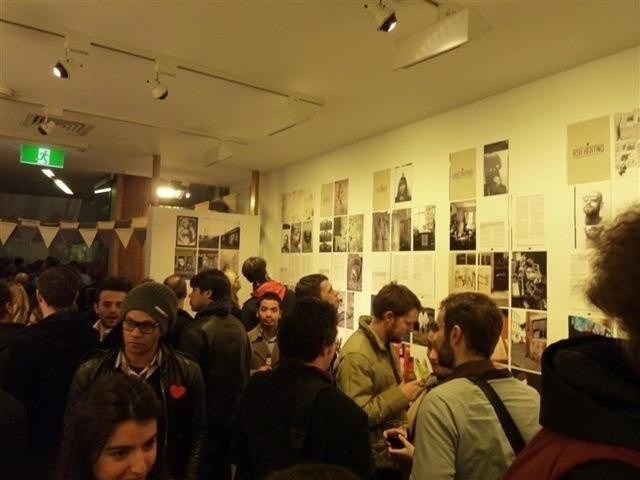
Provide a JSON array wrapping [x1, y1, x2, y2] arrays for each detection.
[[291, 230, 301, 253], [395, 177, 411, 202], [178, 218, 196, 246], [513, 254, 544, 309], [502, 207, 640, 480], [0, 257, 543, 480], [575, 190, 613, 249], [281, 235, 289, 253]]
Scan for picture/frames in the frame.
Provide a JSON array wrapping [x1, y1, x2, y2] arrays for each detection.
[[176, 216, 198, 247]]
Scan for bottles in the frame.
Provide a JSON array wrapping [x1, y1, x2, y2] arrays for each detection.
[[404, 357, 416, 383], [265, 353, 273, 369]]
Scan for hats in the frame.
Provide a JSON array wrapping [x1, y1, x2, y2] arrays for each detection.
[[122, 283, 178, 332]]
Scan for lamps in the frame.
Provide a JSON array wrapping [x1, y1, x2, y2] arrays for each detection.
[[265, 102, 307, 137], [361, 1, 397, 33], [145, 61, 177, 101], [394, 8, 492, 71], [52, 35, 90, 81], [205, 144, 232, 166], [39, 104, 62, 135]]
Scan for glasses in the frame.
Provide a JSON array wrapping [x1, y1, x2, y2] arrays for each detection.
[[122, 321, 154, 334]]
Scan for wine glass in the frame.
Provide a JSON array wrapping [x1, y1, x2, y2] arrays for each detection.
[[387, 420, 404, 472]]
